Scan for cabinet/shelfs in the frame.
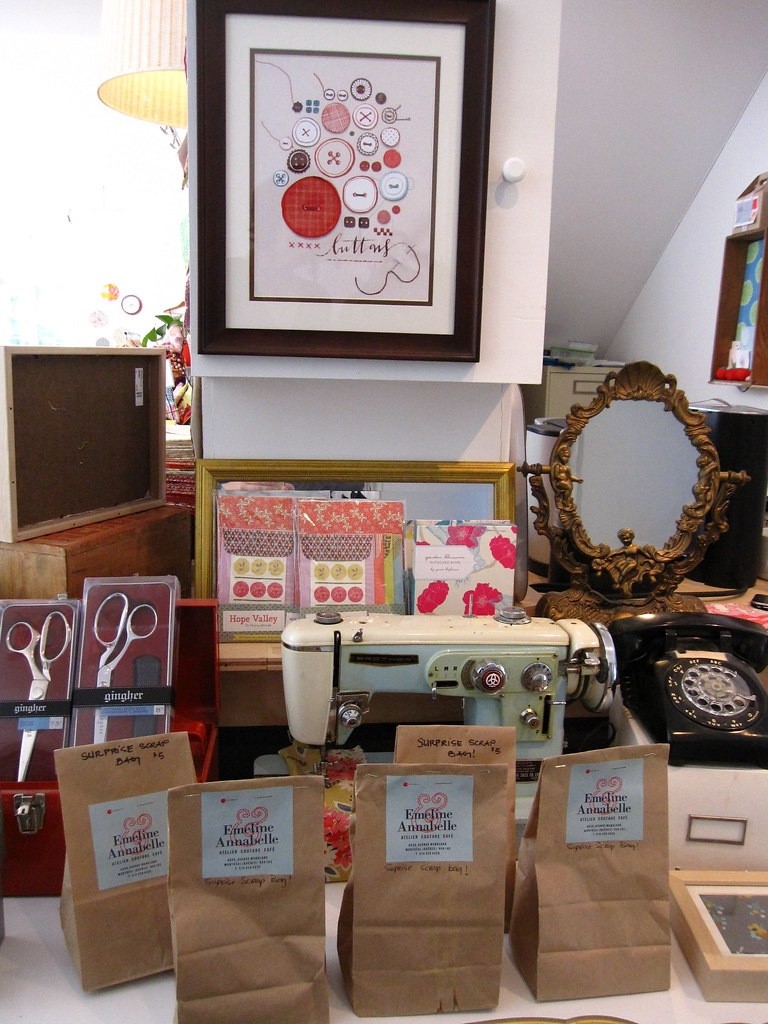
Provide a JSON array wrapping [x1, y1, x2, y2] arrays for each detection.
[[519, 366, 624, 425], [0, 506, 193, 598], [182, 0, 562, 385]]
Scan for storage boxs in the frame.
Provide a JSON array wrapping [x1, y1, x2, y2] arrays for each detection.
[[618, 704, 767, 873], [0, 575, 220, 897], [732, 172, 768, 234]]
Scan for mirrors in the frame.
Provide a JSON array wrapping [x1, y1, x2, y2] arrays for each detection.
[[195, 458, 515, 604], [515, 363, 752, 628]]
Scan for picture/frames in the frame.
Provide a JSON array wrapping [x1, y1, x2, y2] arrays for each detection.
[[196, 0, 497, 363], [669, 869, 768, 1003]]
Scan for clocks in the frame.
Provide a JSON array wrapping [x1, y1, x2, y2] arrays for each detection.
[[121, 295, 142, 315]]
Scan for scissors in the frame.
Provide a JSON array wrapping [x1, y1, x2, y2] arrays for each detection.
[[0, 608, 71, 783], [90, 590, 160, 748]]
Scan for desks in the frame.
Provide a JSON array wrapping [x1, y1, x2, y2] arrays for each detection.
[[0, 860, 767, 1024], [217, 642, 610, 727], [521, 570, 767, 689]]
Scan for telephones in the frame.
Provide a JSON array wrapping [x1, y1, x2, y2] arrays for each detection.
[[607, 609, 768, 767]]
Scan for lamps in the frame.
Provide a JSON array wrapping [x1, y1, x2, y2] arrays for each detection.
[[96, 2, 189, 131]]
